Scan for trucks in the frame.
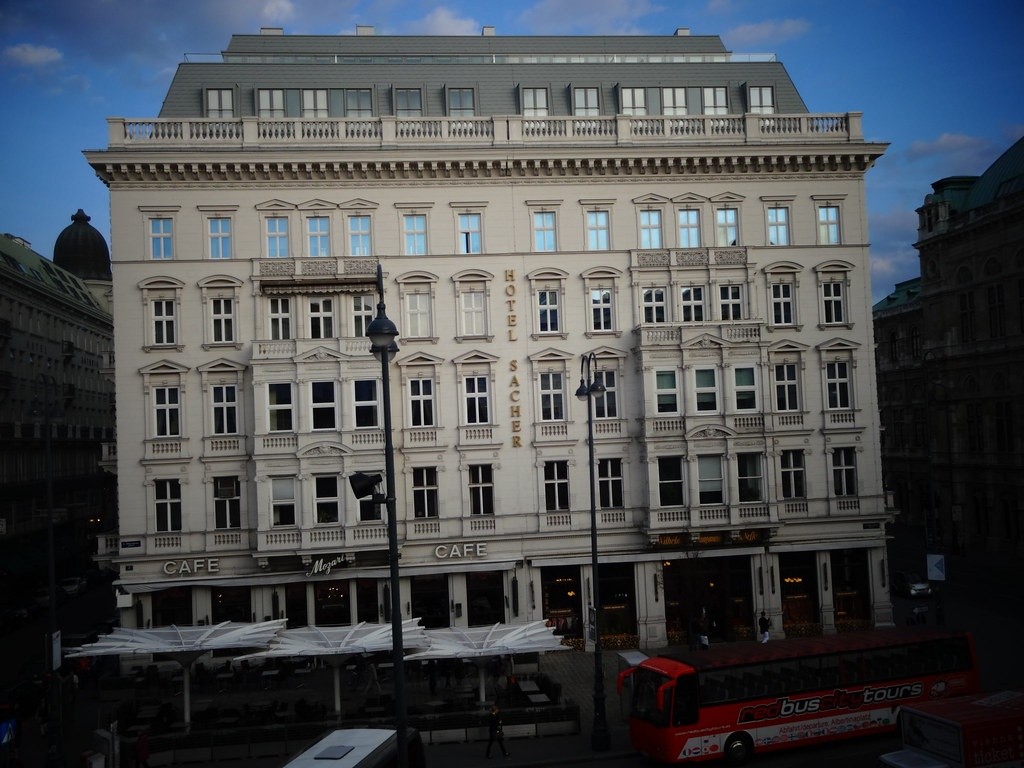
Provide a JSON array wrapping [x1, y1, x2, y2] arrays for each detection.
[[873, 685, 1023, 766]]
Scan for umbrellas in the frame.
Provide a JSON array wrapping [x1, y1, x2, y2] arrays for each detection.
[[62, 616, 573, 732]]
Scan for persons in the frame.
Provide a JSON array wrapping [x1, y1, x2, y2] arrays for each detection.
[[687, 622, 717, 650], [759, 611, 771, 644], [934, 598, 945, 627], [133, 729, 150, 768], [907, 607, 927, 625], [35, 707, 49, 736], [486, 705, 510, 758]]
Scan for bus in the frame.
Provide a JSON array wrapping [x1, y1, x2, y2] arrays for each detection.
[[279, 726, 427, 767], [614, 625, 978, 768]]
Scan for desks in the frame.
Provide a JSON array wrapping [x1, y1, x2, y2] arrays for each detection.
[[122, 677, 553, 732]]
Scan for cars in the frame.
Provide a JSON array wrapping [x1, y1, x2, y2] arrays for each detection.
[[1, 563, 119, 722]]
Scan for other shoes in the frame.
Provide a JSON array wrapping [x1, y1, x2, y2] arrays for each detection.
[[504, 752, 511, 757], [486, 756, 493, 759]]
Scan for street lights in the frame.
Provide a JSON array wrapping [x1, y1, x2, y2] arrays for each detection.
[[576, 352, 616, 750], [932, 398, 969, 552], [365, 265, 412, 766], [32, 373, 69, 658]]
